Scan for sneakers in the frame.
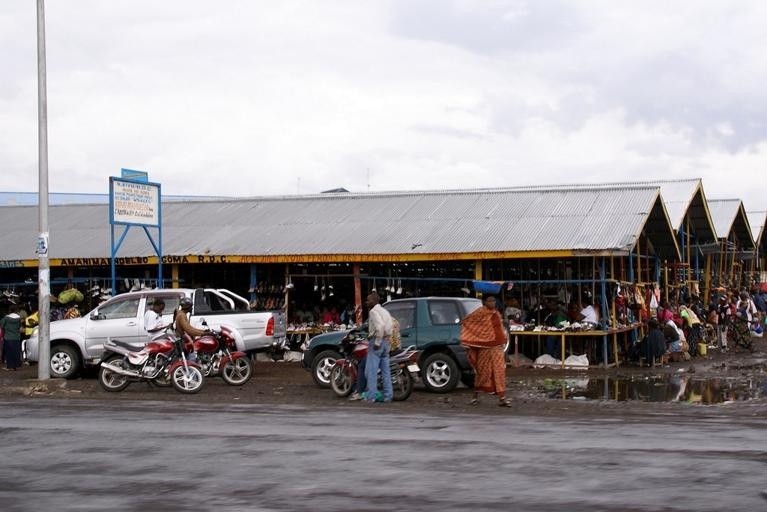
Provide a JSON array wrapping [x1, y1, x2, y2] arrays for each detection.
[[466, 398, 478, 407], [348, 390, 393, 404], [498, 397, 512, 407]]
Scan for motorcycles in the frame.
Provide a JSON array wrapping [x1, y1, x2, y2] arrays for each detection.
[[331, 329, 411, 402], [99, 325, 252, 393]]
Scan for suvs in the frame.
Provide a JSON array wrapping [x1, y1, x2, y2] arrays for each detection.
[[301, 297, 511, 393]]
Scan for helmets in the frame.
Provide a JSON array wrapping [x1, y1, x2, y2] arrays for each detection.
[[180, 298, 193, 309]]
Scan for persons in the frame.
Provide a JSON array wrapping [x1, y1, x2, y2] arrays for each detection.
[[361, 293, 394, 403], [1, 297, 82, 372], [347, 315, 403, 401], [172, 298, 212, 344], [143, 299, 173, 341], [460, 294, 512, 408], [294, 306, 356, 326], [611, 271, 767, 368], [526, 295, 602, 360]]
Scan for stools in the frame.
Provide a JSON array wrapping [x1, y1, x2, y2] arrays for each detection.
[[640, 355, 664, 368]]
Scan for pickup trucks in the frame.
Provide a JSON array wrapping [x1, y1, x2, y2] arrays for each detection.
[[22, 285, 286, 378]]
